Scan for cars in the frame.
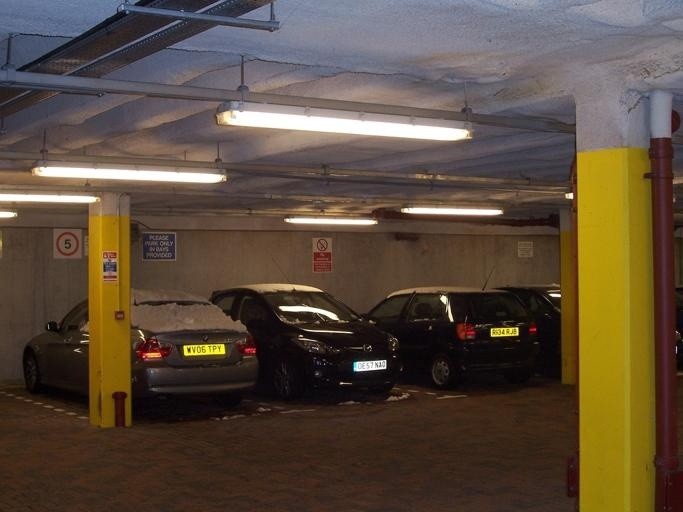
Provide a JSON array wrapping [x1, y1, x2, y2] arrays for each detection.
[[22, 285, 261, 422], [492, 283, 560, 379], [208, 283, 407, 403]]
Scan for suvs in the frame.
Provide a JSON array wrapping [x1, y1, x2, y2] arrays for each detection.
[[359, 285, 544, 392]]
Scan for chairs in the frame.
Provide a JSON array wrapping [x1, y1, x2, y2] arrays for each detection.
[[412, 303, 433, 320]]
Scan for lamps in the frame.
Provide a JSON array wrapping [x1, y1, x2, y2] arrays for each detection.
[[0, 178, 101, 218], [214, 55, 474, 141], [29, 129, 227, 183], [283, 215, 379, 225], [401, 208, 504, 216]]
